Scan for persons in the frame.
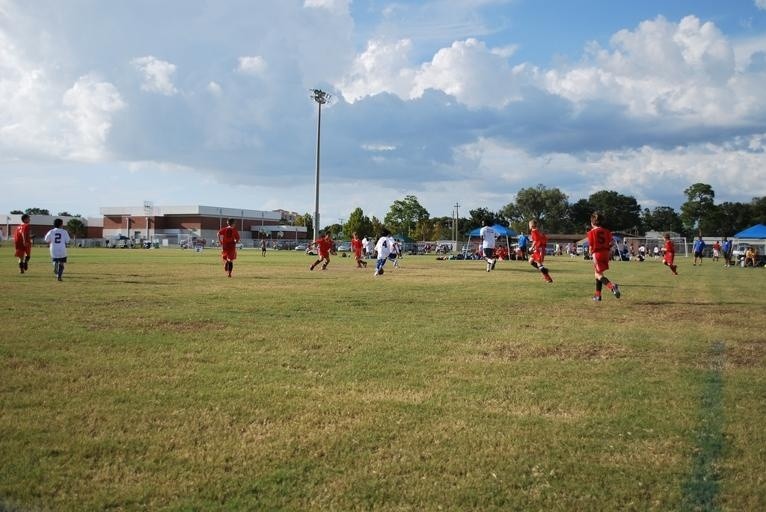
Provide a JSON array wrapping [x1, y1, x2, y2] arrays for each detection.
[[305, 244, 314, 255], [365, 239, 373, 259], [45, 219, 71, 282], [496, 246, 517, 261], [260, 236, 269, 257], [712, 241, 721, 262], [691, 235, 705, 266], [372, 232, 390, 277], [612, 242, 663, 262], [389, 236, 400, 269], [745, 247, 755, 267], [723, 237, 731, 268], [528, 219, 554, 284], [455, 244, 483, 259], [361, 235, 368, 255], [14, 214, 31, 274], [351, 232, 368, 269], [518, 231, 530, 260], [588, 211, 621, 302], [480, 221, 501, 272], [663, 233, 678, 276], [217, 218, 241, 278], [398, 239, 404, 259], [310, 231, 338, 271], [555, 242, 589, 261]]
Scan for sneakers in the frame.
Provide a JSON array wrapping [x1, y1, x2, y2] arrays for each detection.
[[321, 266, 327, 270], [53, 261, 58, 274], [364, 261, 367, 268], [612, 284, 620, 298], [18, 267, 25, 274], [541, 267, 549, 275], [227, 271, 232, 277], [224, 260, 229, 272], [23, 262, 28, 271], [542, 279, 552, 283], [592, 295, 601, 301], [56, 274, 63, 281], [356, 264, 362, 268], [490, 259, 496, 270], [309, 265, 314, 272], [487, 269, 490, 272]]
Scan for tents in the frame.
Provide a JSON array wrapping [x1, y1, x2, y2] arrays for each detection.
[[734, 223, 766, 266], [464, 224, 517, 261]]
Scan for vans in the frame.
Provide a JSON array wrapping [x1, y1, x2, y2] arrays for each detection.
[[235, 240, 244, 249], [462, 244, 477, 252], [436, 242, 453, 251], [733, 245, 752, 256]]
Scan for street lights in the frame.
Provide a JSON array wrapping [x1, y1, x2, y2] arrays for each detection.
[[311, 89, 332, 244], [453, 202, 462, 252], [307, 88, 330, 247]]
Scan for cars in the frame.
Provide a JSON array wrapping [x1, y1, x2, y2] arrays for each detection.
[[307, 248, 318, 255], [295, 244, 307, 251], [338, 242, 351, 251], [182, 241, 205, 250]]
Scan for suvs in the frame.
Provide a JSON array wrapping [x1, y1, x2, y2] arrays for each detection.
[[418, 244, 427, 252], [576, 245, 584, 254], [545, 243, 555, 255], [180, 237, 206, 247]]
